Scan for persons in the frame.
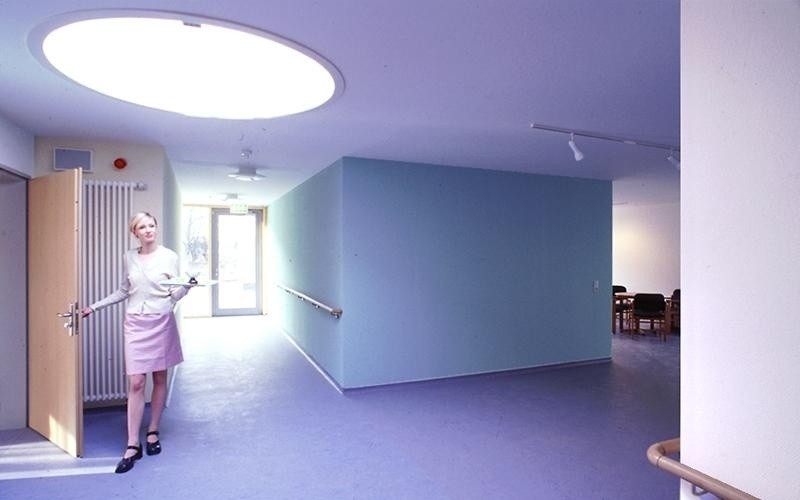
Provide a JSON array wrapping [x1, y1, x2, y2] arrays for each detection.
[[82, 212, 197, 474]]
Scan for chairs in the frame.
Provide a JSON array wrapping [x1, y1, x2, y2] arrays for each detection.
[[612, 285, 680, 344]]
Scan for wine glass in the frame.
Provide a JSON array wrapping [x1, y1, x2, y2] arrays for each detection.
[[186, 272, 200, 284]]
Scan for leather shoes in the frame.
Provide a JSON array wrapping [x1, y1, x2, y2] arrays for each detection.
[[145, 430, 162, 456], [115, 443, 143, 475]]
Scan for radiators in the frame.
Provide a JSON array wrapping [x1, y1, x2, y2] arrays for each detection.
[[81, 179, 147, 403]]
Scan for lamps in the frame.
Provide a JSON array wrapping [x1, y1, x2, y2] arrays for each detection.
[[531, 124, 679, 170], [228, 167, 267, 182]]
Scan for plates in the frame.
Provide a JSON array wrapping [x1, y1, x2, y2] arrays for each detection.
[[159, 277, 220, 287]]
